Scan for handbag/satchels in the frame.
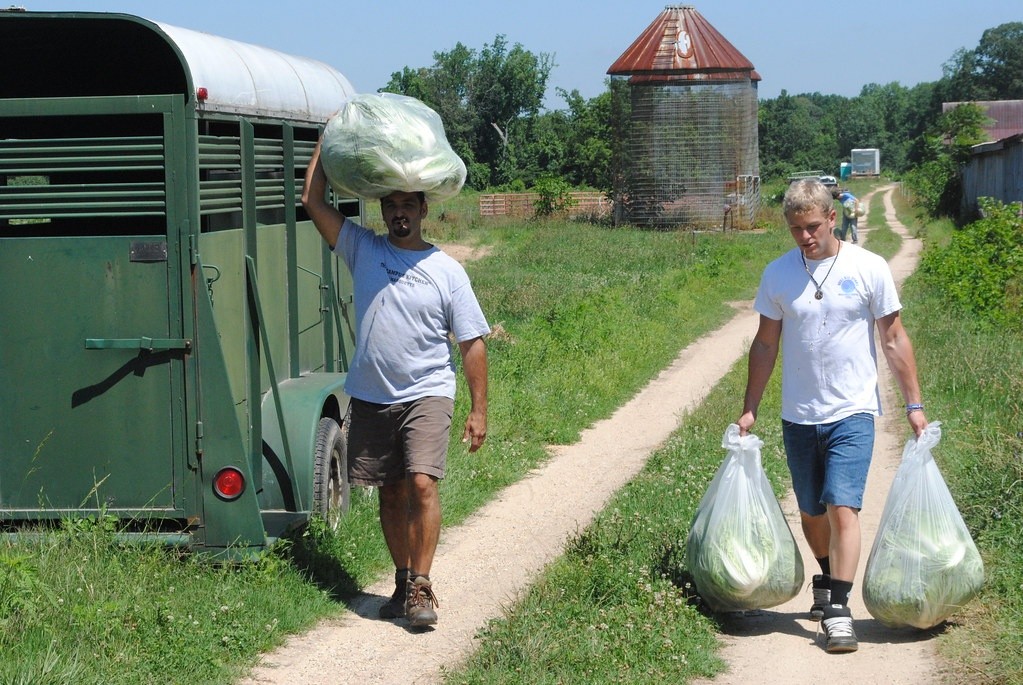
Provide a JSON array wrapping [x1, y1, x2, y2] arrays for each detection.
[[862, 420, 987, 629], [320, 94, 468, 202], [842, 201, 866, 219], [683, 422, 806, 610]]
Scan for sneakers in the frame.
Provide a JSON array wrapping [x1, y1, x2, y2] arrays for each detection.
[[821, 604, 858, 651], [379, 569, 412, 619], [406, 574, 439, 626], [810, 574, 831, 620]]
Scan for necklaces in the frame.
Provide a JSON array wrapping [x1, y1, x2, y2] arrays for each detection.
[[801, 237, 841, 299]]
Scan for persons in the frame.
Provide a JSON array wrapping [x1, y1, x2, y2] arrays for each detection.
[[831, 191, 861, 243], [736, 175, 930, 651], [304, 108, 491, 630]]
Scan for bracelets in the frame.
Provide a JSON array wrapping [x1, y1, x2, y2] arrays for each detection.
[[906, 403, 925, 415]]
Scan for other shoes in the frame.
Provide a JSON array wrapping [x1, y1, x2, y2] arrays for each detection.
[[852, 240, 859, 244]]
